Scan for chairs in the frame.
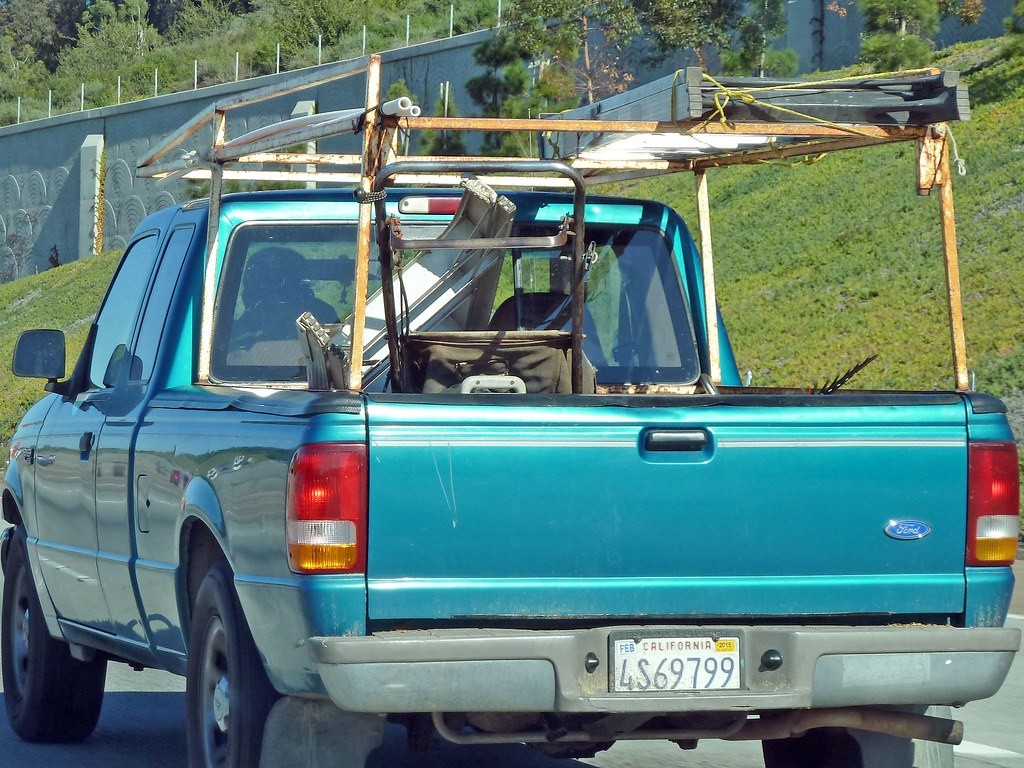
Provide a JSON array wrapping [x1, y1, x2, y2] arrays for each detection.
[[234, 296, 346, 346], [491, 289, 604, 366]]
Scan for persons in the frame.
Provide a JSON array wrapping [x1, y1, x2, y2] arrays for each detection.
[[229, 246, 315, 332]]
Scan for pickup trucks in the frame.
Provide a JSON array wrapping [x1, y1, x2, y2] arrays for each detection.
[[0, 188, 1021, 768]]
